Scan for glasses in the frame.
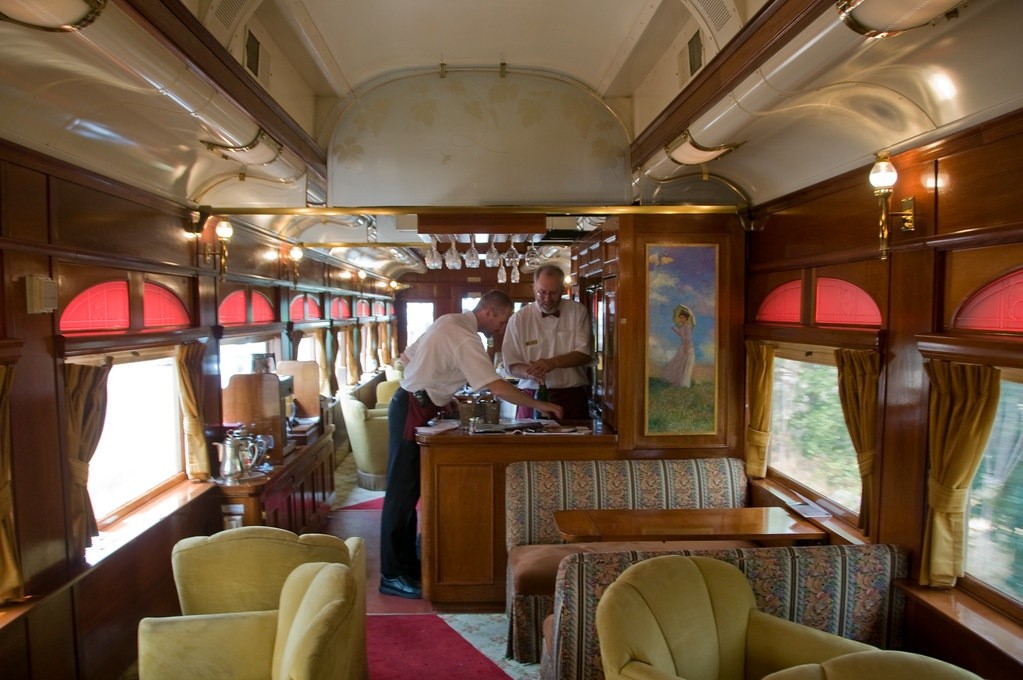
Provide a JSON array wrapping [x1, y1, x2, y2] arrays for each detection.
[[535, 286, 561, 298]]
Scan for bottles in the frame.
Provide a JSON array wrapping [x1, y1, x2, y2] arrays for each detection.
[[592, 405, 603, 434]]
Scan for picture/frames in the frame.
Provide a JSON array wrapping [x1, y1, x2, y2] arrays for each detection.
[[644, 243, 719, 436]]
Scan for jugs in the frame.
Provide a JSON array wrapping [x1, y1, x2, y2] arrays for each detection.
[[211, 427, 258, 482]]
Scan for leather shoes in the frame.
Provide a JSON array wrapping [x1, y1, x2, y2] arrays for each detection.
[[379, 574, 421, 598]]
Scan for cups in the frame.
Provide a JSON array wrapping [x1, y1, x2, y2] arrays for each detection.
[[457, 400, 476, 432], [483, 401, 501, 424]]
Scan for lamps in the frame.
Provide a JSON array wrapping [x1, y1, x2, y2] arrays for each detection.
[[204, 218, 233, 276], [868, 150, 915, 262], [283, 244, 304, 286]]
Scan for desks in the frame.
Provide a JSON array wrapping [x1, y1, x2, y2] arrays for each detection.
[[218, 426, 337, 535], [554, 506, 826, 549]]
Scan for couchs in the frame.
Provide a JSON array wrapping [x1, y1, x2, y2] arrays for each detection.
[[540, 542, 912, 680], [595, 554, 882, 680], [762, 650, 984, 680], [137, 562, 358, 680], [375, 379, 400, 408], [503, 457, 748, 665], [339, 394, 388, 491], [170, 525, 367, 616]]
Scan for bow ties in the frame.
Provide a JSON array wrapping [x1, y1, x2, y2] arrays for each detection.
[[542, 310, 560, 318]]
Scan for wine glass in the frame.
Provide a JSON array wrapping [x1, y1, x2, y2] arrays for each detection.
[[253, 434, 274, 472], [424, 235, 540, 283]]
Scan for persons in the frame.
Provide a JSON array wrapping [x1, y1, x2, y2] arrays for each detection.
[[500, 265, 595, 429], [379, 291, 563, 599]]
